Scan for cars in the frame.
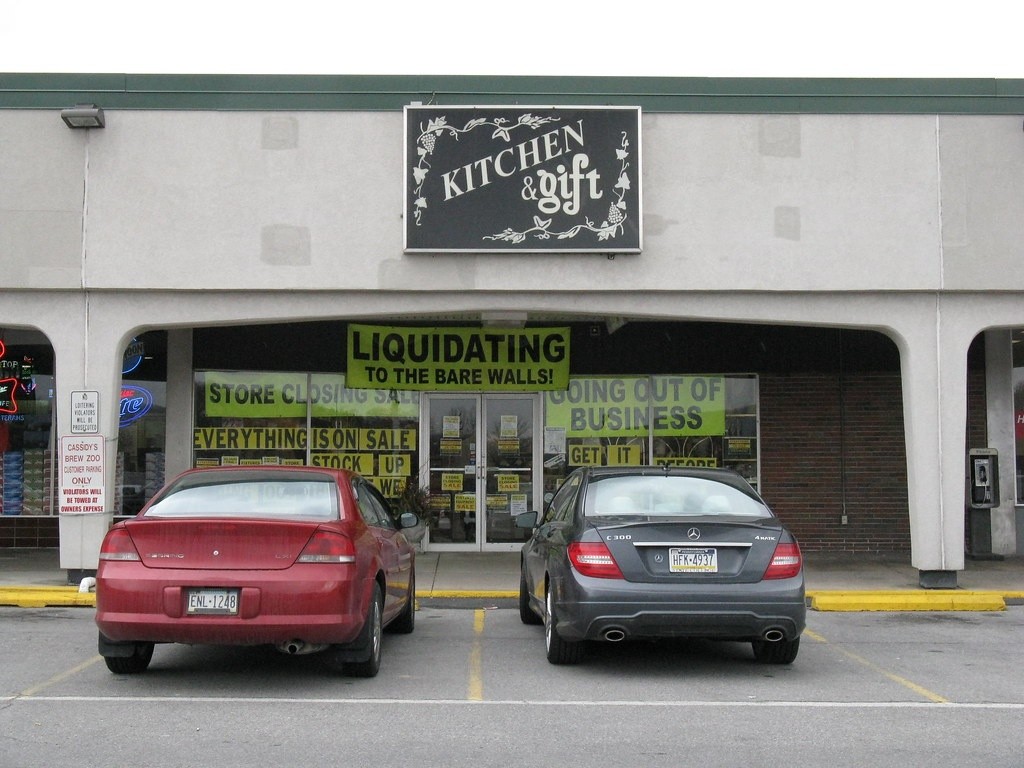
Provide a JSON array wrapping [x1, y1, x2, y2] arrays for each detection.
[[95, 464, 420, 678], [438, 490, 476, 540], [517, 462, 806, 665]]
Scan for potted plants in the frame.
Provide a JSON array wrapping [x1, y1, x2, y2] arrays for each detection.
[[388, 462, 436, 554]]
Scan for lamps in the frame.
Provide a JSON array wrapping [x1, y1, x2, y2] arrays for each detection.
[[60, 103, 106, 129]]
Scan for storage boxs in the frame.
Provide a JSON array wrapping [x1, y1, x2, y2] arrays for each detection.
[[0, 449, 59, 514], [114, 451, 168, 517]]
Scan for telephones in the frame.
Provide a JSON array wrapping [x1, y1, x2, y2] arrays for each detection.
[[975, 459, 990, 486]]
